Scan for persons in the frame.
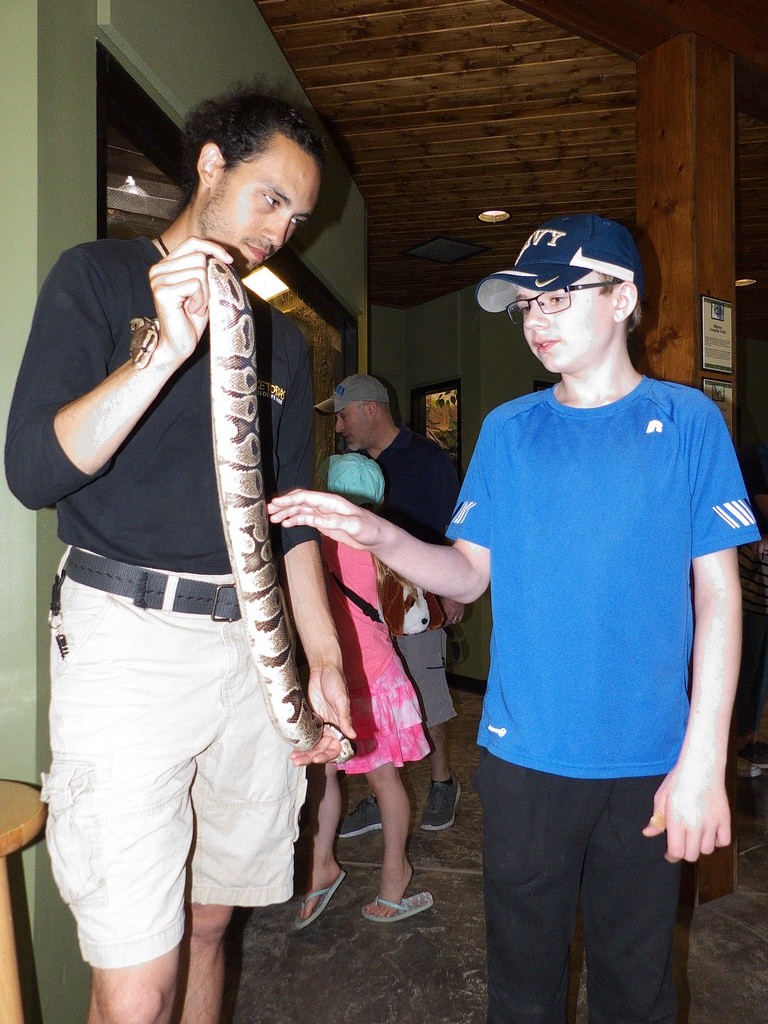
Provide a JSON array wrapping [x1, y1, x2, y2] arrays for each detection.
[[311, 371, 462, 839], [3, 76, 355, 1017], [293, 452, 433, 927], [265, 211, 761, 1024]]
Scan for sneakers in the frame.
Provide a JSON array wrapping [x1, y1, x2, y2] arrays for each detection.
[[420, 772, 461, 830], [736, 758, 762, 777], [736, 741, 768, 768], [335, 796, 383, 838]]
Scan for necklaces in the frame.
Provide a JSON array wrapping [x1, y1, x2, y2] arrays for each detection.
[[155, 233, 170, 258]]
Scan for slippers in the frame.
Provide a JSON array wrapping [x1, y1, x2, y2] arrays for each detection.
[[361, 892, 433, 923], [294, 870, 346, 930]]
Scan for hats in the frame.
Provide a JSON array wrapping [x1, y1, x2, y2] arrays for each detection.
[[474, 213, 645, 314], [315, 453, 386, 511], [312, 374, 389, 415]]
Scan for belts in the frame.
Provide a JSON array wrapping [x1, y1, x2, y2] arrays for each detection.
[[63, 545, 244, 624]]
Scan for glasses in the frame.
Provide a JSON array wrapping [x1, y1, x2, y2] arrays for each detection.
[[427, 627, 461, 670], [506, 279, 625, 325]]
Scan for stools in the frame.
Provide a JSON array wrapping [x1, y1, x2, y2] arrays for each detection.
[[0, 781, 46, 1023]]
